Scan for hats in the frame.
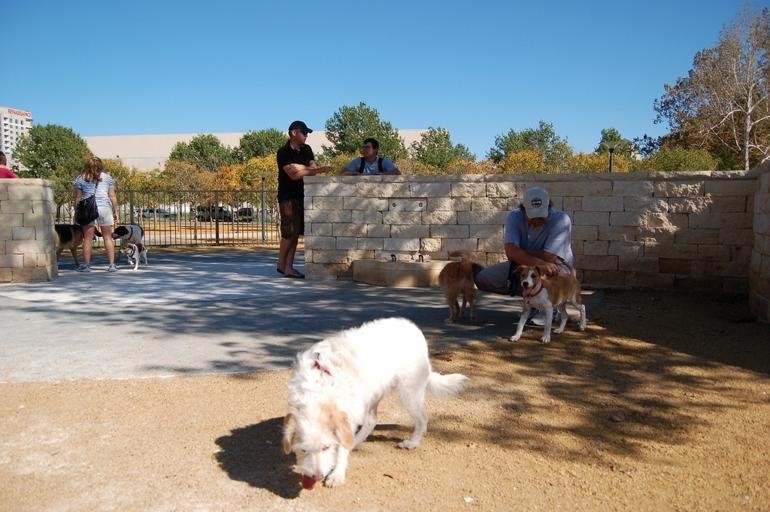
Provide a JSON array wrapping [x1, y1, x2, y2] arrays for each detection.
[[523, 188, 550, 218], [289, 121, 312, 133]]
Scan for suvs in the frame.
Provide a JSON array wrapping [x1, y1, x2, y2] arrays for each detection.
[[196, 205, 233, 223], [133, 208, 177, 221], [233, 208, 255, 223]]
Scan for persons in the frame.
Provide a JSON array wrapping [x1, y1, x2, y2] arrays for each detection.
[[74, 156, 119, 272], [475, 186, 576, 326], [276, 120, 332, 278], [0, 151, 20, 178], [339, 138, 402, 175]]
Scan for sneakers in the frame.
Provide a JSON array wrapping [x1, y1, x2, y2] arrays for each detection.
[[526, 308, 558, 325], [109, 264, 116, 272], [77, 264, 90, 271]]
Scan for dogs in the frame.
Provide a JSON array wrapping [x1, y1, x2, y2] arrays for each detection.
[[508, 256, 587, 345], [109, 223, 144, 243], [438, 250, 488, 324], [279, 316, 472, 491], [55, 223, 103, 267], [118, 242, 148, 269]]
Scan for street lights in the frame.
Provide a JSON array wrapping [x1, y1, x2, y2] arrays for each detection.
[[600, 140, 624, 172]]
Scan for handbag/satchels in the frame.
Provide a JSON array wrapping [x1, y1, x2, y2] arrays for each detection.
[[75, 195, 99, 225], [276, 264, 305, 278]]
